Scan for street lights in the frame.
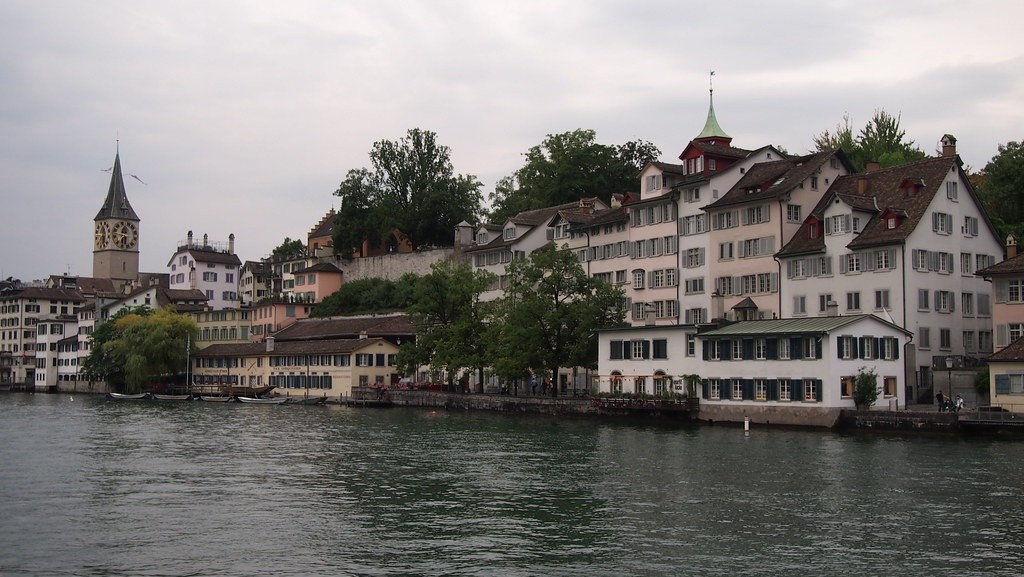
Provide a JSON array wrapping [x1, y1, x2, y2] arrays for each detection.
[[945, 354, 954, 412]]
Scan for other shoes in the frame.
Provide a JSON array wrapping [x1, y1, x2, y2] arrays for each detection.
[[543, 395, 546, 397], [533, 394, 535, 396]]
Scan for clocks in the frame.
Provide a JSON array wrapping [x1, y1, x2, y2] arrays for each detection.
[[95, 220, 111, 250], [111, 220, 138, 250]]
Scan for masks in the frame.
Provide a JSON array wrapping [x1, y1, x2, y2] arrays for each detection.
[[957, 398, 959, 400]]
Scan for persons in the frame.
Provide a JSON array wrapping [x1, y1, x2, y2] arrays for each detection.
[[936, 390, 963, 412], [540, 379, 552, 394], [530, 378, 538, 395]]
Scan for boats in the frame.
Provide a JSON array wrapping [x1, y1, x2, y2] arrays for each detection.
[[201, 396, 231, 402], [219, 386, 276, 396], [296, 397, 329, 405], [239, 397, 288, 404], [109, 392, 146, 399], [154, 393, 190, 399]]
